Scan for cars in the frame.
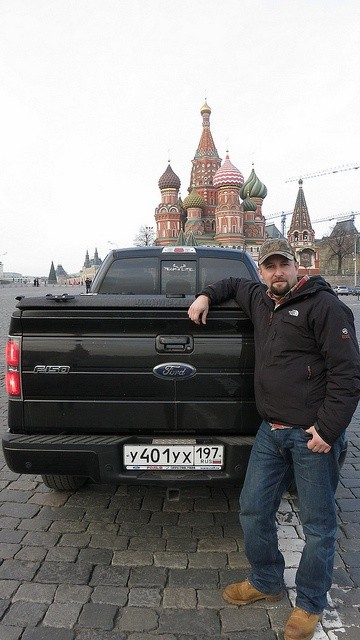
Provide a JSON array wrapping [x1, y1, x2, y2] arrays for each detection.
[[353, 287, 360, 296], [348, 288, 353, 294]]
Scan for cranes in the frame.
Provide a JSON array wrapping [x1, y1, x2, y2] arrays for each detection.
[[286, 162, 359, 184], [264, 209, 294, 236], [311, 210, 360, 224]]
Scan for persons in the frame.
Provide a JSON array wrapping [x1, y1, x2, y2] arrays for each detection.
[[85, 277, 92, 294], [187, 238, 360, 640]]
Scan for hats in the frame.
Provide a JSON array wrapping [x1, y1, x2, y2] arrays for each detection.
[[259, 239, 295, 265]]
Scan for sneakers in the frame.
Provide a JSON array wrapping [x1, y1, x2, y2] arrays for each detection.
[[222, 577, 282, 606], [284, 606, 323, 640]]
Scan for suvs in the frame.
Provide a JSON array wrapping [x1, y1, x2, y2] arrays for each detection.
[[333, 285, 348, 295]]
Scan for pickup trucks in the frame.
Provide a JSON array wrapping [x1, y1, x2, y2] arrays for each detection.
[[2, 245, 347, 490]]
